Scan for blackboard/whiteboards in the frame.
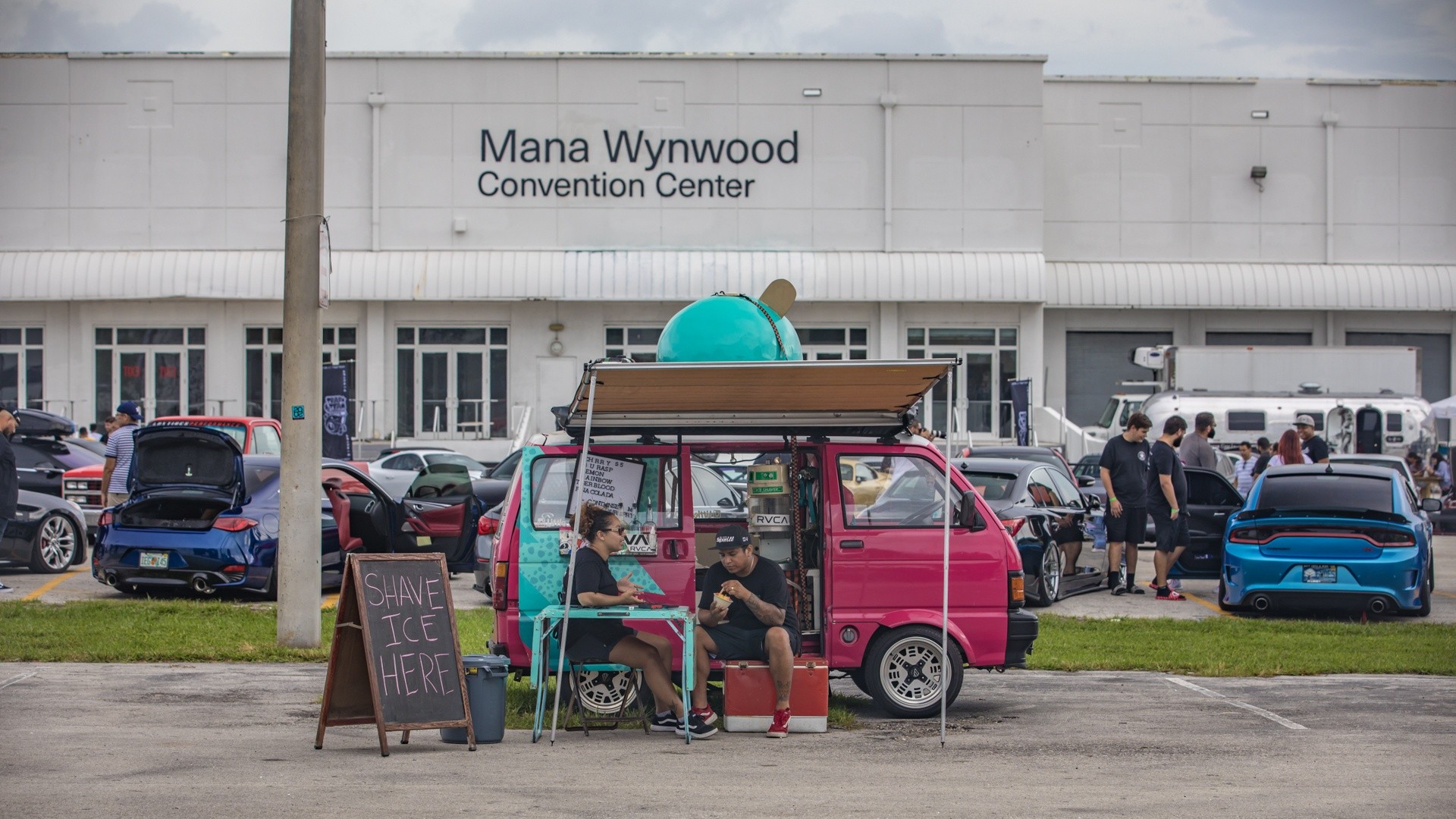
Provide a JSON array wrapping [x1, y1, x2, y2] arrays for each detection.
[[324, 554, 470, 730]]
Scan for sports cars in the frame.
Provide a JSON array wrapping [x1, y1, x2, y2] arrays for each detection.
[[91, 424, 491, 603], [854, 456, 1127, 608], [1164, 461, 1443, 620]]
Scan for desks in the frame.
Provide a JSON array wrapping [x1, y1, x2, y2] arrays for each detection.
[[527, 605, 695, 744]]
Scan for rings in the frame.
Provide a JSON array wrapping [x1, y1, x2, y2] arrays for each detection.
[[731, 587, 734, 590]]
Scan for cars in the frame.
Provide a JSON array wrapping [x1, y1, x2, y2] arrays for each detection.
[[366, 443, 490, 505], [471, 453, 751, 605], [0, 396, 1456, 618]]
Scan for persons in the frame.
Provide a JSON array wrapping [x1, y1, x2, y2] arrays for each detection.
[[1234, 440, 1258, 501], [79, 427, 96, 441], [101, 401, 145, 509], [1250, 437, 1271, 482], [1098, 412, 1153, 594], [98, 417, 116, 445], [1403, 450, 1452, 508], [1267, 429, 1313, 468], [556, 501, 718, 739], [0, 404, 19, 593], [1292, 415, 1330, 464], [691, 524, 800, 737], [89, 423, 103, 442], [910, 421, 935, 442], [1179, 411, 1218, 471], [1147, 415, 1190, 601]]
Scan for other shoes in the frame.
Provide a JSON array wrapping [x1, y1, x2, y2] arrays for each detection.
[[0, 582, 13, 592], [1169, 579, 1183, 589], [1213, 586, 1219, 594]]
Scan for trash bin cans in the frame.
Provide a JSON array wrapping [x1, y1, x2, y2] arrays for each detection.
[[441, 656, 508, 744]]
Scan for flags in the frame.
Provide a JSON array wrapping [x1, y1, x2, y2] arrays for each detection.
[[1011, 383, 1029, 447], [1109, 497, 1116, 502], [1171, 509, 1180, 513]]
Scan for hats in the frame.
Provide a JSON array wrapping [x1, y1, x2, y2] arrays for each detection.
[[1293, 414, 1316, 426], [708, 525, 753, 550], [117, 402, 144, 420], [2, 406, 22, 425]]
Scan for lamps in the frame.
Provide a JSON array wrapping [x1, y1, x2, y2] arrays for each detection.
[[1250, 166, 1268, 190], [803, 87, 822, 97], [549, 339, 565, 356], [1252, 110, 1269, 118]]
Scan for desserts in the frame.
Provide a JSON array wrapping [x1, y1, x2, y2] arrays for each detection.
[[714, 593, 733, 610]]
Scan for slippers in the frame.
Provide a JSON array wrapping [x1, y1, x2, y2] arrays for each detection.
[[1149, 582, 1177, 591], [1111, 585, 1128, 595], [1126, 585, 1145, 594], [1156, 590, 1186, 601]]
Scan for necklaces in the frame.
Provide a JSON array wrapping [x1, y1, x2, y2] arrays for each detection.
[[590, 544, 602, 552], [750, 556, 754, 572]]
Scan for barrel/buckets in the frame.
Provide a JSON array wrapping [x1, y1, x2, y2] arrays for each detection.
[[440, 655, 510, 742]]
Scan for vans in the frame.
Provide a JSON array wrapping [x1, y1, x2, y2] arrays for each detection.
[[480, 352, 1041, 721]]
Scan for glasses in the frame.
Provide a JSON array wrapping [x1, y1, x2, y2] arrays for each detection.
[[1243, 461, 1246, 469], [1210, 423, 1216, 427], [596, 528, 625, 536], [115, 414, 124, 416]]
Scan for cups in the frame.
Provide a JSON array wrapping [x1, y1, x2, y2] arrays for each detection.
[[713, 593, 733, 610]]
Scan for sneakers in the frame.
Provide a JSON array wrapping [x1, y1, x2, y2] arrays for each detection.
[[675, 710, 719, 738], [691, 704, 718, 725], [649, 710, 678, 731], [767, 708, 791, 737]]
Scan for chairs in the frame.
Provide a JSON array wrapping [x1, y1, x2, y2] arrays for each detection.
[[323, 477, 363, 550], [567, 663, 651, 738]]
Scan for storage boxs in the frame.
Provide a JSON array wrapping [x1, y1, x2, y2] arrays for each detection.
[[719, 658, 829, 734]]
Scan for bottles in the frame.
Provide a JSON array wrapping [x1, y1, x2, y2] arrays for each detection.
[[602, 495, 609, 511], [642, 496, 656, 534], [628, 497, 642, 534], [617, 496, 630, 534]]
